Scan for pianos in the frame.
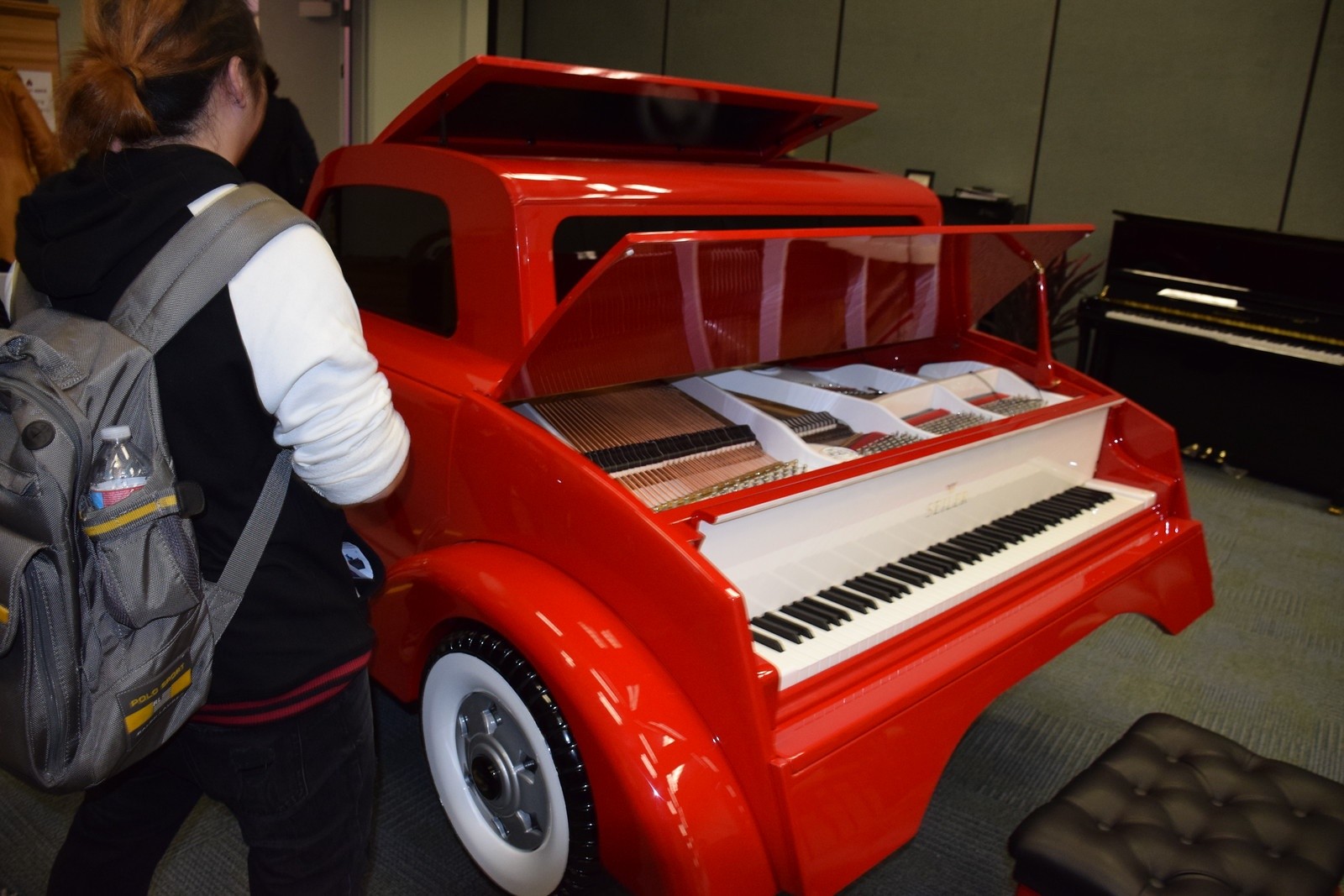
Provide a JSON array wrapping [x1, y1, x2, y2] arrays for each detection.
[[1075, 209, 1344, 515], [502, 349, 1158, 692]]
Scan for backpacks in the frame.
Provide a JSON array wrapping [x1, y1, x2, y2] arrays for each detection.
[[0, 184, 296, 798]]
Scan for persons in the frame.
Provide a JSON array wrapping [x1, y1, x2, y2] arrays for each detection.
[[0, 59, 318, 336], [12, 0, 412, 896]]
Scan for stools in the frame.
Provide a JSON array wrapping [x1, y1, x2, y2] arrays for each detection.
[[1004, 713, 1344, 896]]
